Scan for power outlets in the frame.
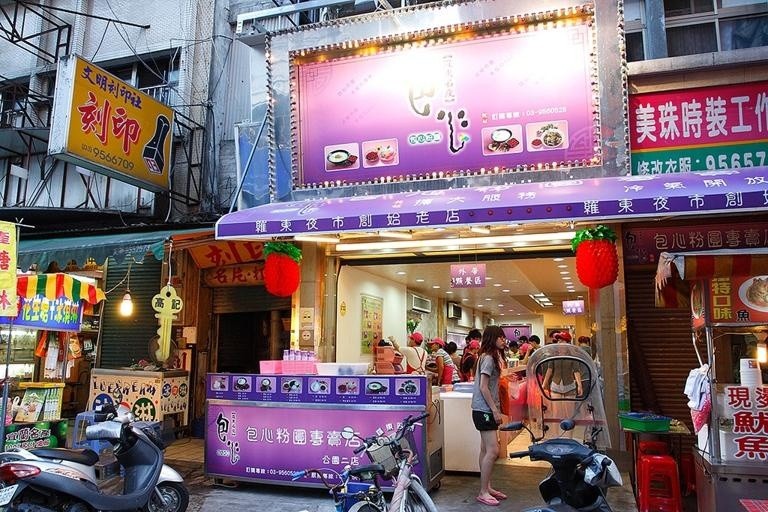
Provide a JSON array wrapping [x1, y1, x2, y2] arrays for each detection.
[[299, 329, 314, 346], [300, 308, 315, 323]]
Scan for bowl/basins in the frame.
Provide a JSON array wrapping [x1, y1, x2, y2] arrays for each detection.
[[313, 362, 371, 377], [740, 358, 762, 386], [259, 360, 283, 376]]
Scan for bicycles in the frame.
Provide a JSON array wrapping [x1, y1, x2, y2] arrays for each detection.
[[291, 468, 348, 512], [337, 413, 437, 512]]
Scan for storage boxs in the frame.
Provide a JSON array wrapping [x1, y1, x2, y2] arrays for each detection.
[[313, 364, 369, 375], [259, 361, 281, 374]]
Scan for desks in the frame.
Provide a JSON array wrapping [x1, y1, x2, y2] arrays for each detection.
[[623, 421, 691, 487]]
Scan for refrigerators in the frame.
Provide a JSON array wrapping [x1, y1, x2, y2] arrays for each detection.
[[1, 381, 65, 423]]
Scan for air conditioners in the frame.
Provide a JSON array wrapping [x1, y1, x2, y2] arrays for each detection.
[[407, 294, 431, 314], [448, 303, 462, 319]]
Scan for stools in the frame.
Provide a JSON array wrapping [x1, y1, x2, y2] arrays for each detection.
[[73, 410, 112, 454], [638, 456, 681, 512], [638, 441, 667, 460]]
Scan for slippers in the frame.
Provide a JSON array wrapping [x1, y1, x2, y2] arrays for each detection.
[[475, 496, 500, 506], [491, 492, 507, 500]]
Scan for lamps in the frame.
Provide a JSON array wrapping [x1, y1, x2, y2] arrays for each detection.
[[754, 335, 767, 364], [120, 275, 133, 317]]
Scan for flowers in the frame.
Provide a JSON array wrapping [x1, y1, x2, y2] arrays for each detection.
[[406, 318, 421, 334]]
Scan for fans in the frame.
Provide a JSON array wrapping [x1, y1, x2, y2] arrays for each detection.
[[148, 335, 179, 368]]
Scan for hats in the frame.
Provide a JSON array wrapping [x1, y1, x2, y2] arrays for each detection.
[[408, 333, 423, 343], [522, 344, 530, 352], [428, 338, 445, 346], [556, 332, 572, 340], [470, 341, 479, 350]]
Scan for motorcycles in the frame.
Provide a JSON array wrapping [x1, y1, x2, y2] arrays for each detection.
[[0, 413, 189, 512], [500, 420, 622, 512]]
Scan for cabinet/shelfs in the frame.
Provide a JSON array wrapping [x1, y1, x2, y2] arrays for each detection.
[[440, 369, 529, 472], [375, 345, 404, 374], [205, 373, 445, 493], [33, 295, 105, 411]]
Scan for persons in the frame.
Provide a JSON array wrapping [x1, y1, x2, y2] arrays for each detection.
[[527, 335, 540, 351], [388, 333, 427, 375], [549, 329, 560, 341], [460, 341, 479, 381], [518, 336, 526, 345], [510, 340, 520, 352], [470, 326, 507, 506], [428, 338, 461, 386], [520, 343, 537, 364], [578, 336, 598, 417], [446, 341, 461, 370], [540, 331, 583, 438], [469, 330, 481, 343]]
